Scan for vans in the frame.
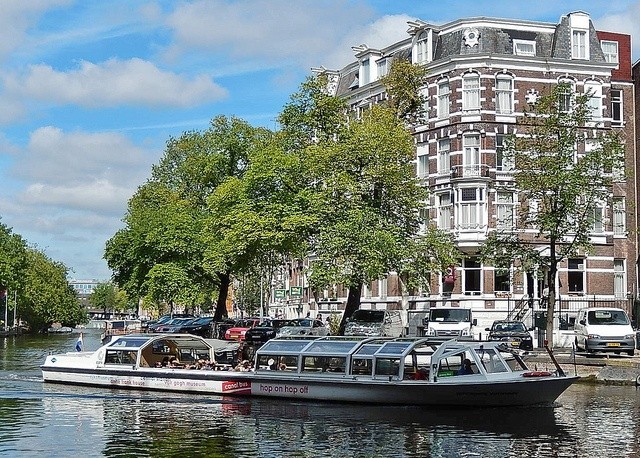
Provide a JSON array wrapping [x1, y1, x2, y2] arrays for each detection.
[[343, 309, 403, 336], [574, 306, 637, 356]]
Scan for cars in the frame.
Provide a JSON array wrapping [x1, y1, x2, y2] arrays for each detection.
[[245, 319, 291, 340], [276, 318, 332, 335], [148, 316, 171, 332], [485, 319, 533, 350], [180, 316, 214, 337], [153, 317, 194, 332], [224, 318, 260, 340]]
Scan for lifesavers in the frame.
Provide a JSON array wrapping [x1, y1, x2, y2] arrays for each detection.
[[523, 372, 551, 377]]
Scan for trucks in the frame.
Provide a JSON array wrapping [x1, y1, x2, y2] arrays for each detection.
[[422, 306, 477, 337]]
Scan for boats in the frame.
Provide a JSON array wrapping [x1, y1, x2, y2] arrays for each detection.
[[41, 333, 580, 404]]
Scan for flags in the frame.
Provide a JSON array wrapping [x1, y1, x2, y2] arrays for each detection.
[[75, 333, 83, 351]]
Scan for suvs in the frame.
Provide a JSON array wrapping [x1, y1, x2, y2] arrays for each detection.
[[141, 313, 195, 327]]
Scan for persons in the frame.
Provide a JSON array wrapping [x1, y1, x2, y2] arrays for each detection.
[[155, 356, 286, 372]]
